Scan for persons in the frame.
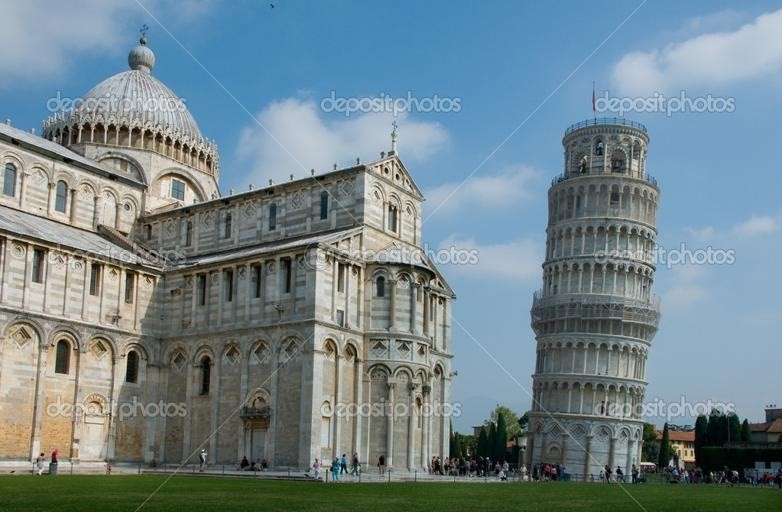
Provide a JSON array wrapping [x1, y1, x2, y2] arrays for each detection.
[[240, 455, 249, 469], [341, 453, 349, 474], [198, 448, 209, 472], [254, 458, 264, 471], [330, 456, 342, 481], [52, 448, 60, 462], [350, 452, 362, 477], [36, 452, 48, 475], [261, 458, 267, 468], [376, 453, 386, 478], [312, 458, 322, 478]]
